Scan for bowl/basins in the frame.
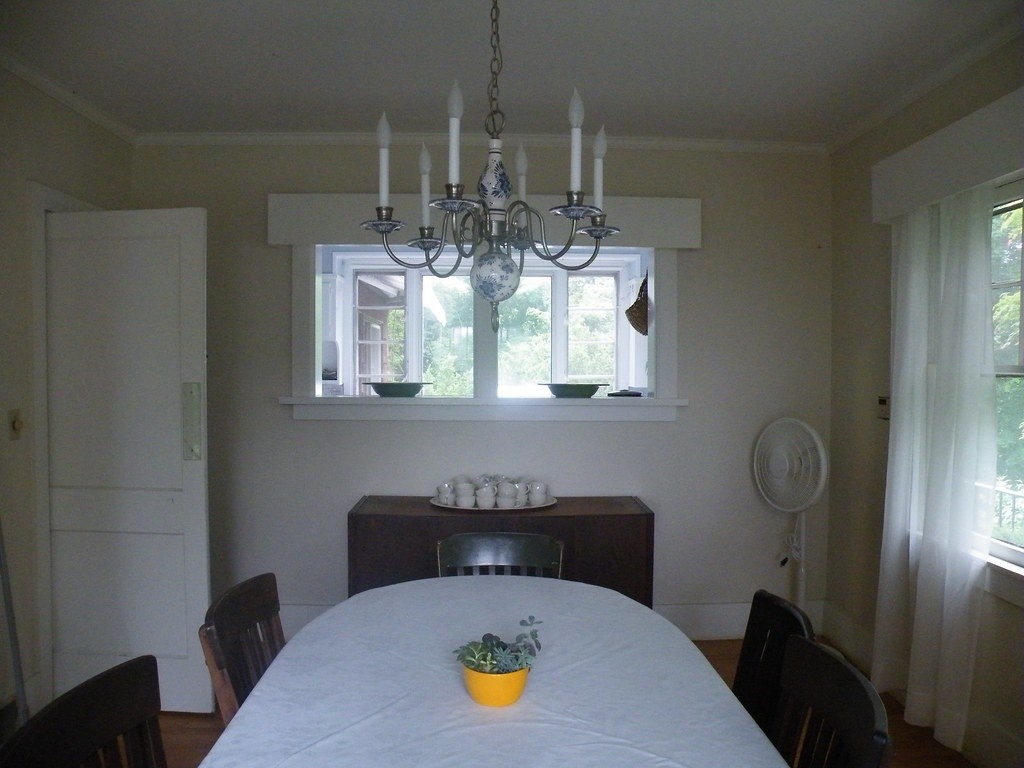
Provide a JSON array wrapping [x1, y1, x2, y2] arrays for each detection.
[[538, 383, 610, 398], [363, 382, 432, 397]]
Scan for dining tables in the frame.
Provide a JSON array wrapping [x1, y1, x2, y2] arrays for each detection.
[[194, 575, 792, 767]]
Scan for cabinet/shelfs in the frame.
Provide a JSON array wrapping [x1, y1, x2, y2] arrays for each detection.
[[347, 496, 654, 610]]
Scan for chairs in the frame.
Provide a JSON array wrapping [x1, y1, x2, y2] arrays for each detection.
[[199, 573, 287, 727], [0, 656, 168, 768], [437, 532, 565, 578], [733, 588, 888, 768]]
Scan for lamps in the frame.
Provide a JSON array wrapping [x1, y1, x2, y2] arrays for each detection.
[[362, 0, 621, 328]]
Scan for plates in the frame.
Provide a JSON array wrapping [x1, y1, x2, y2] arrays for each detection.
[[429, 497, 558, 511]]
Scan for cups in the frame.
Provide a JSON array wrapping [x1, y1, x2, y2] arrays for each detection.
[[434, 474, 551, 508]]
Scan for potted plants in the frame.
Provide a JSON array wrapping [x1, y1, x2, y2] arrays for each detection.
[[453, 615, 543, 706]]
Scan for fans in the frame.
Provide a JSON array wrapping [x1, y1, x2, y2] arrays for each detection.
[[753, 417, 829, 610]]
